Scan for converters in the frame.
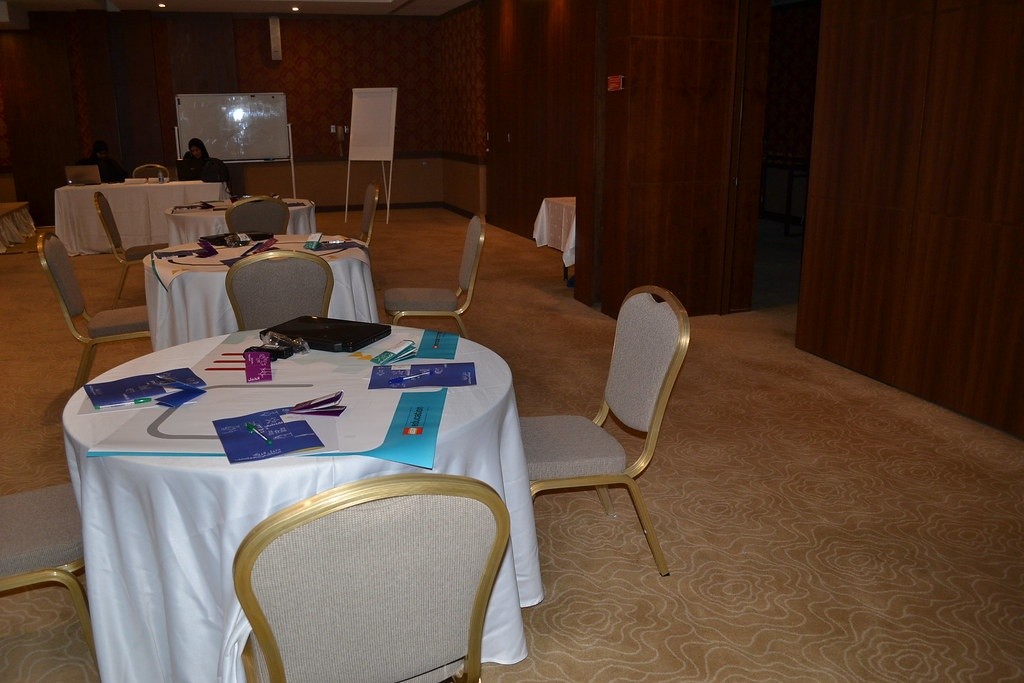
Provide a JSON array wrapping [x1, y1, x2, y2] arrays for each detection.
[[261, 344, 293, 358]]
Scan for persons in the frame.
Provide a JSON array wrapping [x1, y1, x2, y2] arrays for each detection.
[[179, 138, 217, 182], [88, 140, 113, 180]]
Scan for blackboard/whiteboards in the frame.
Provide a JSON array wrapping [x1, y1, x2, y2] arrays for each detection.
[[176, 92, 291, 163]]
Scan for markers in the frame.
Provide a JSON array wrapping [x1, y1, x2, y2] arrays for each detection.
[[264, 158, 275, 161]]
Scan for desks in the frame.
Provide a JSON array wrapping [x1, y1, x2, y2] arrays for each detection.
[[54, 182, 231, 255], [62, 324, 542, 683], [531, 197, 577, 282], [0, 202, 36, 253], [142, 234, 380, 350], [164, 198, 317, 247]]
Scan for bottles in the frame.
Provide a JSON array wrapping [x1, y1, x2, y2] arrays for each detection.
[[158, 171, 163, 184]]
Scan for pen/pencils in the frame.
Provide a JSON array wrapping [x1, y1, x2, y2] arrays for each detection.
[[322, 240, 346, 243], [96, 398, 151, 409], [247, 422, 271, 444], [159, 255, 187, 260], [212, 208, 228, 211], [388, 371, 432, 384]]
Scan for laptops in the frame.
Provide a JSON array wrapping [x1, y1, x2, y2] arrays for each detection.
[[260, 316, 391, 352], [199, 231, 273, 245], [64, 165, 101, 184]]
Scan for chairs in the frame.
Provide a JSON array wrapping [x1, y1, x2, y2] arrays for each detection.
[[513, 284, 690, 577], [226, 197, 289, 237], [132, 165, 170, 181], [384, 215, 485, 339], [93, 191, 172, 300], [0, 482, 99, 668], [357, 186, 378, 248], [232, 474, 511, 683], [226, 250, 334, 333], [38, 229, 152, 403]]
